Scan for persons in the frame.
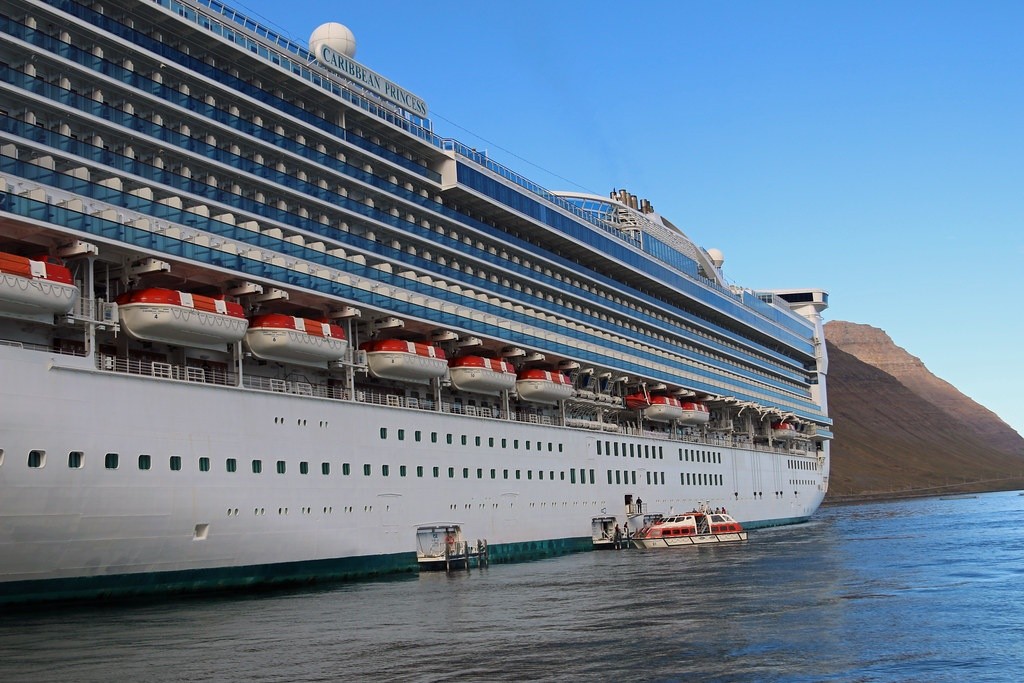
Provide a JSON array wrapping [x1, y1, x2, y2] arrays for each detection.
[[658, 514, 663, 522], [692, 505, 726, 514], [636, 497, 642, 513], [615, 522, 627, 536]]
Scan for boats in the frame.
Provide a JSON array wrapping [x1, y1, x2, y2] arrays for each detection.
[[359, 339, 448, 387], [114, 287, 250, 356], [643, 394, 683, 425], [515, 368, 572, 405], [446, 355, 518, 398], [772, 422, 796, 441], [631, 498, 749, 549], [247, 314, 347, 370], [0, 0, 835, 606], [0, 252, 80, 317], [677, 401, 710, 425]]
